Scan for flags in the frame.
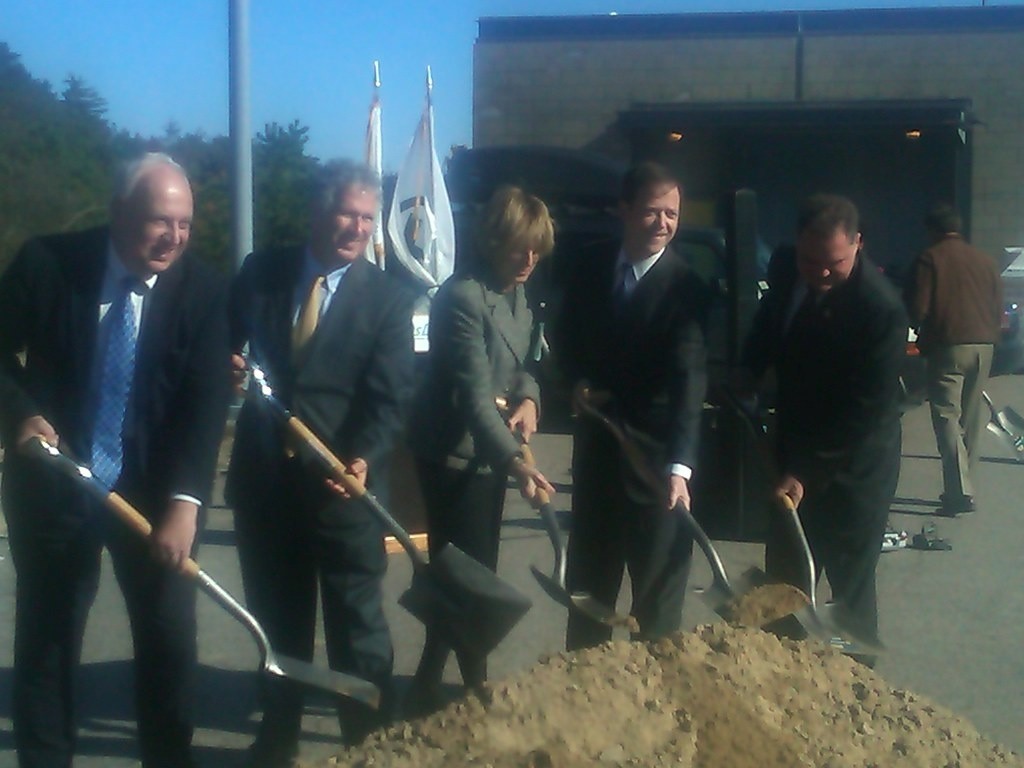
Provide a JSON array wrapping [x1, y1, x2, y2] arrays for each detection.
[[390, 104, 453, 299], [362, 100, 385, 274]]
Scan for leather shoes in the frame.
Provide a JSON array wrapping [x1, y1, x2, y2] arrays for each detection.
[[936, 496, 975, 517], [940, 494, 974, 503]]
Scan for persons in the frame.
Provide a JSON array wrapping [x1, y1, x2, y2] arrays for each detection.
[[1, 154, 238, 767], [406, 185, 557, 720], [223, 158, 417, 767], [550, 162, 707, 651], [908, 203, 1003, 517], [736, 193, 907, 665]]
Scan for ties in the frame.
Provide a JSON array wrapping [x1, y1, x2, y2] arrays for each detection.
[[608, 264, 634, 315], [790, 283, 821, 342], [280, 278, 328, 455], [90, 276, 137, 494]]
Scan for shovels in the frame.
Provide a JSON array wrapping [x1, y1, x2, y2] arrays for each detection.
[[491, 393, 636, 632], [26, 431, 383, 716], [574, 391, 828, 652], [237, 351, 532, 663], [720, 387, 888, 662], [980, 389, 1024, 464]]
